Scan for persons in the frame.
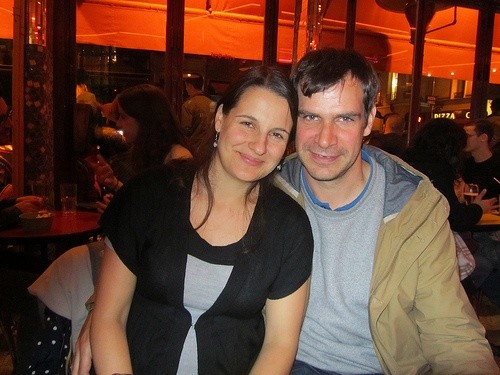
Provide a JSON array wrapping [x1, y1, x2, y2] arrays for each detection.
[[485, 97, 500, 150], [368, 114, 406, 157], [402, 118, 500, 315], [0, 40, 128, 161], [0, 95, 44, 353], [90, 64, 314, 375], [70, 48, 500, 375], [180, 73, 217, 157], [27, 84, 193, 375]]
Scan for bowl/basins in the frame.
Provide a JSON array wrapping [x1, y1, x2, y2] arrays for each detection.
[[20, 210, 52, 233]]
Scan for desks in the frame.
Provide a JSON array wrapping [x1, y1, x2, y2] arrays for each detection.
[[0, 211, 102, 319], [454, 200, 500, 261]]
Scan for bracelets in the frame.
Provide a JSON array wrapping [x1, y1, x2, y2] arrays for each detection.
[[107, 176, 118, 190]]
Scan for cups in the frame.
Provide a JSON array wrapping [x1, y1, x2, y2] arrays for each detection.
[[463, 184, 479, 206], [60, 183, 77, 214]]
